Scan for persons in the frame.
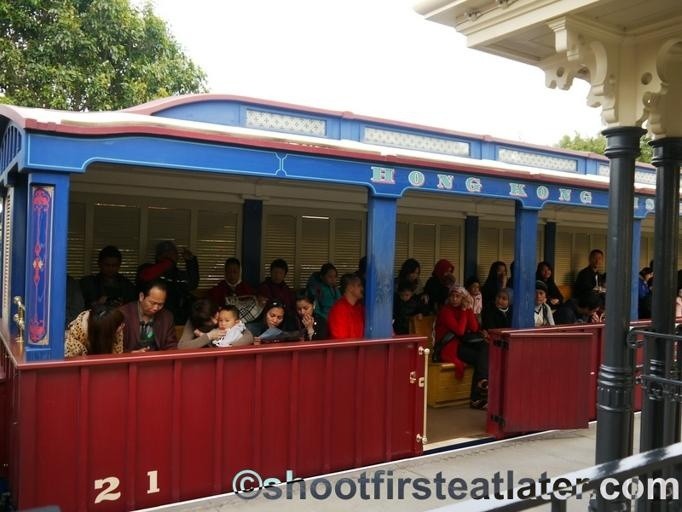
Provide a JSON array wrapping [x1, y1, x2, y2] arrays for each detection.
[[64, 242, 682, 410]]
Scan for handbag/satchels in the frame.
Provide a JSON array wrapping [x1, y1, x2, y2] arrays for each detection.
[[458, 334, 488, 367], [225, 297, 263, 323]]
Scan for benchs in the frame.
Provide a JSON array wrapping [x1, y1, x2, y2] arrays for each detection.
[[412, 308, 472, 407]]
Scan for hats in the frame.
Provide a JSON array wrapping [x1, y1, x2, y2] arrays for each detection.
[[535, 280, 548, 293]]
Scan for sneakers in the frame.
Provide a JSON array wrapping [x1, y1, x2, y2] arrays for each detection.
[[473, 376, 488, 393], [471, 399, 487, 411]]
